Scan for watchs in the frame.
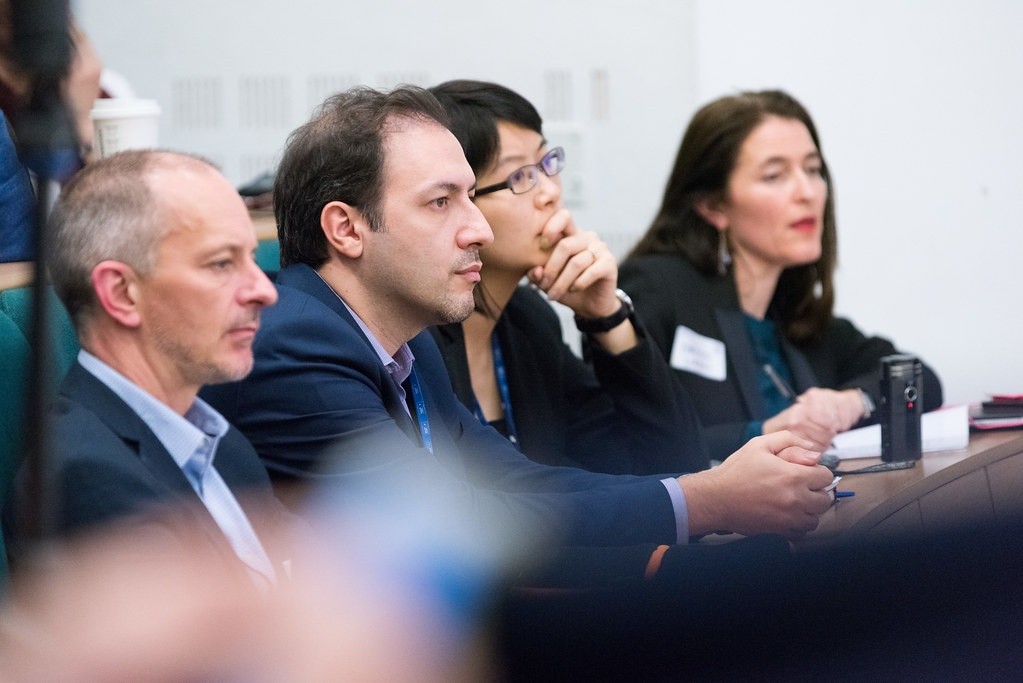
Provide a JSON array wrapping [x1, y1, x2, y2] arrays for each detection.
[[573, 288, 635, 335]]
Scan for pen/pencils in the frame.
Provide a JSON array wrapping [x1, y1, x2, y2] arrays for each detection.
[[763, 363, 840, 449]]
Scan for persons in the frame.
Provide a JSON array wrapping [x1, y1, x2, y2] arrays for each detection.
[[612, 88, 946, 472], [404, 79, 711, 515], [0, 1, 121, 265], [197, 84, 840, 593], [36, 146, 346, 627]]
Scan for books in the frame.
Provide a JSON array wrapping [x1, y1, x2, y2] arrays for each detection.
[[802, 402, 970, 458], [966, 389, 1022, 429]]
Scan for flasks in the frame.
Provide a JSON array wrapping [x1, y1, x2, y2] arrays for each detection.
[[881, 354, 922, 461]]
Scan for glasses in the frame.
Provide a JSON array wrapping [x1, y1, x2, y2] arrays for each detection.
[[475, 144, 566, 198]]
